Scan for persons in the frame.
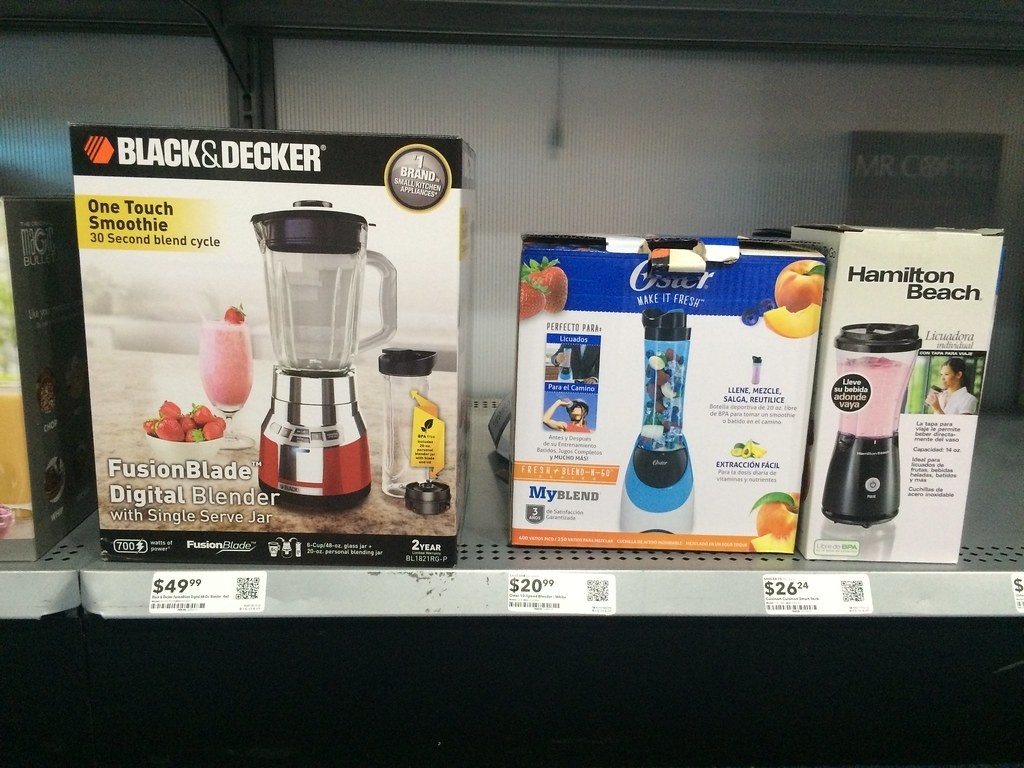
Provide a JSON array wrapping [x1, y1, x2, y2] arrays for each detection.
[[550, 344, 600, 383], [544, 399, 594, 432], [924, 358, 978, 415]]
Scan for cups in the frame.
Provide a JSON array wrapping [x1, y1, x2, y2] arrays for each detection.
[[925, 384, 943, 409], [377, 347, 438, 499], [564, 398, 574, 412], [752, 354, 761, 387]]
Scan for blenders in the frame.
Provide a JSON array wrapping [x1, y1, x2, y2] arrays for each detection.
[[618, 306, 696, 533], [820, 322, 922, 529], [558, 347, 573, 383], [251, 200, 398, 512]]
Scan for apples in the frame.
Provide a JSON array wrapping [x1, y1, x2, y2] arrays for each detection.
[[762, 260, 825, 339], [748, 492, 800, 553]]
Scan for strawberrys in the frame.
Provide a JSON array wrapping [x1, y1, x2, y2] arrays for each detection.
[[143, 402, 226, 441], [224, 307, 245, 324], [519, 255, 568, 321]]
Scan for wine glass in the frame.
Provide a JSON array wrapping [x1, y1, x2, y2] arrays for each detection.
[[198, 320, 256, 451]]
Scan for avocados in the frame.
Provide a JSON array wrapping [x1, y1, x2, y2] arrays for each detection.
[[731, 440, 765, 458]]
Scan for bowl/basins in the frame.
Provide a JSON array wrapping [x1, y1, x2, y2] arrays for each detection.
[[146, 432, 226, 473]]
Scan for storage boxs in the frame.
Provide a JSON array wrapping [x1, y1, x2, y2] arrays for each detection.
[[0, 199, 98, 562], [71, 123, 476, 567], [792, 227, 1008, 564], [507, 234, 828, 557]]
